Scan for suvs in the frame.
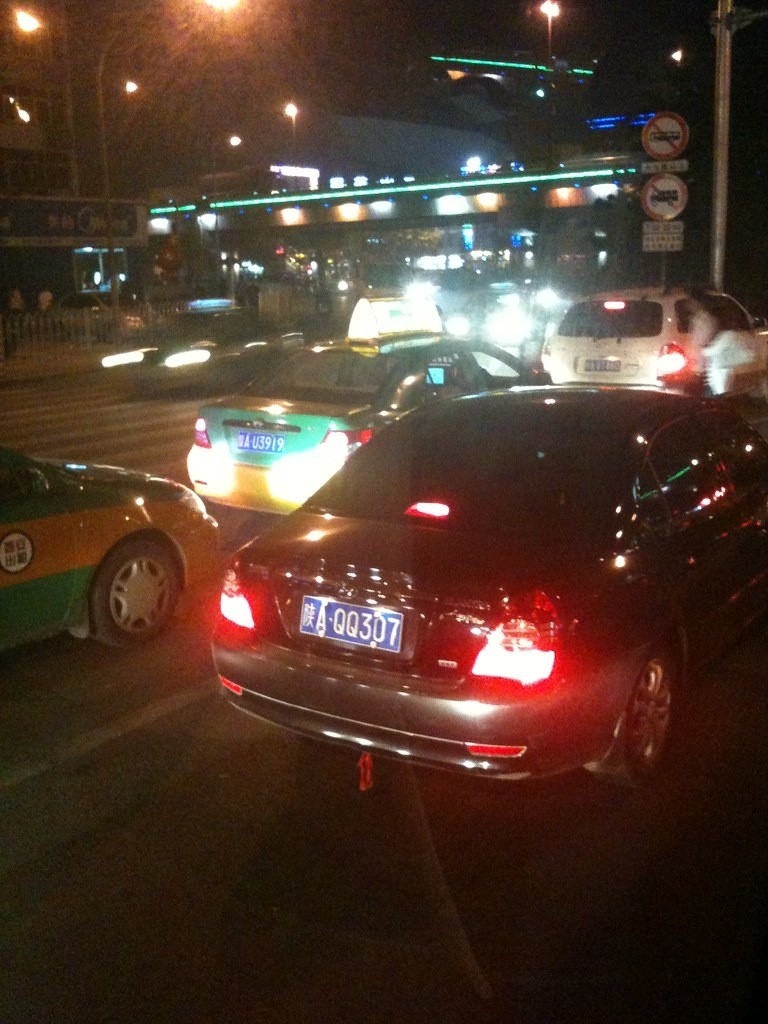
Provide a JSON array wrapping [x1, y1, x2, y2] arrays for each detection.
[[544, 283, 768, 395]]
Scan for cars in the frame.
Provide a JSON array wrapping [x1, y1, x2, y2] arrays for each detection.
[[186, 291, 551, 518], [50, 291, 162, 338], [100, 297, 310, 394], [206, 383, 768, 796], [0, 444, 218, 657]]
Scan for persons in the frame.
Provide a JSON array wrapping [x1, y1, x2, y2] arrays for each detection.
[[316, 283, 332, 328], [685, 288, 757, 398], [235, 273, 260, 308], [8, 286, 26, 313], [38, 287, 53, 312]]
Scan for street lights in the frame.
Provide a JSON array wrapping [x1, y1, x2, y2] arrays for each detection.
[[97, 1, 244, 346], [286, 102, 299, 190], [537, 0, 561, 166]]
